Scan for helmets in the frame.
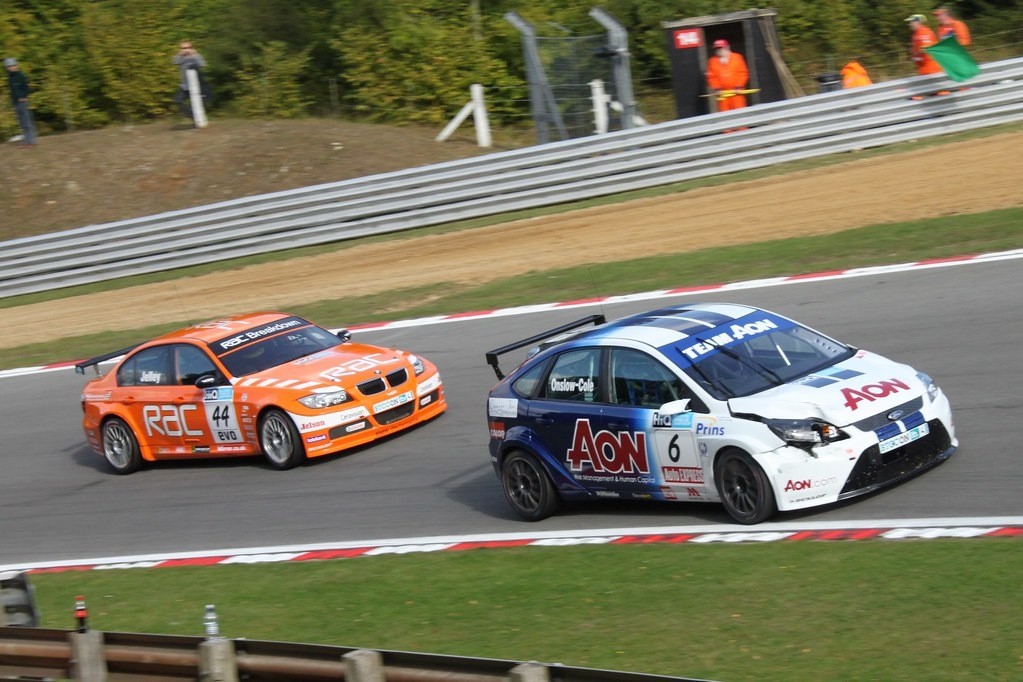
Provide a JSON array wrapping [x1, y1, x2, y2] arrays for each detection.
[[240, 343, 274, 369]]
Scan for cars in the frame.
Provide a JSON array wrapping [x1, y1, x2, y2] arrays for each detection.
[[75, 311, 448, 475], [485, 302, 961, 526]]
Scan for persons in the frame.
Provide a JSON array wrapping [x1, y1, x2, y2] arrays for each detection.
[[705, 39, 749, 133], [840, 5, 973, 153], [621, 352, 716, 409], [172, 39, 209, 124], [2, 58, 40, 145], [239, 343, 279, 376]]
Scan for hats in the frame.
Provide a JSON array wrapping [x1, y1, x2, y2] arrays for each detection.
[[712, 39, 730, 50], [903, 14, 927, 24], [931, 4, 952, 16]]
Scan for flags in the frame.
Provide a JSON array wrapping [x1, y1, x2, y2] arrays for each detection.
[[921, 35, 981, 82]]
